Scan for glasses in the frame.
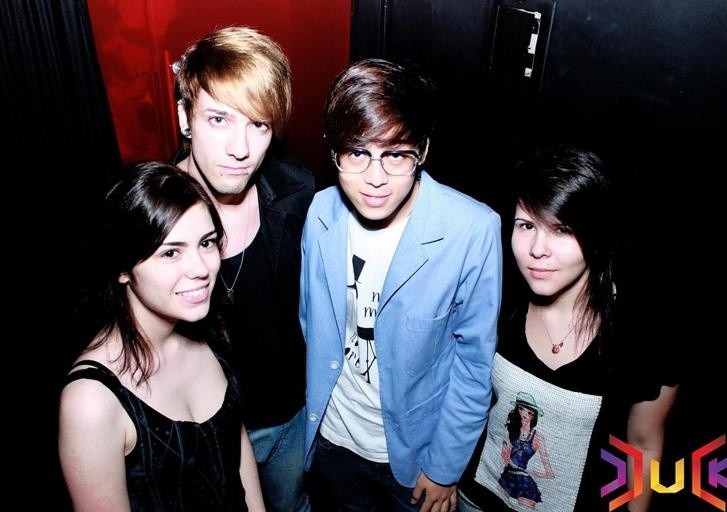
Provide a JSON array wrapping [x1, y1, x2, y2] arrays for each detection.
[[330, 137, 430, 175]]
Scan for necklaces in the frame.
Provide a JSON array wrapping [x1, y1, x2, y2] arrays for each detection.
[[537, 299, 589, 353], [187, 157, 249, 305]]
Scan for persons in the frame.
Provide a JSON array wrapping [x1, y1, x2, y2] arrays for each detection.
[[501, 440, 512, 468], [498, 392, 554, 511], [171, 21, 315, 512], [58, 160, 265, 512], [454, 151, 690, 512], [298, 58, 502, 512]]
